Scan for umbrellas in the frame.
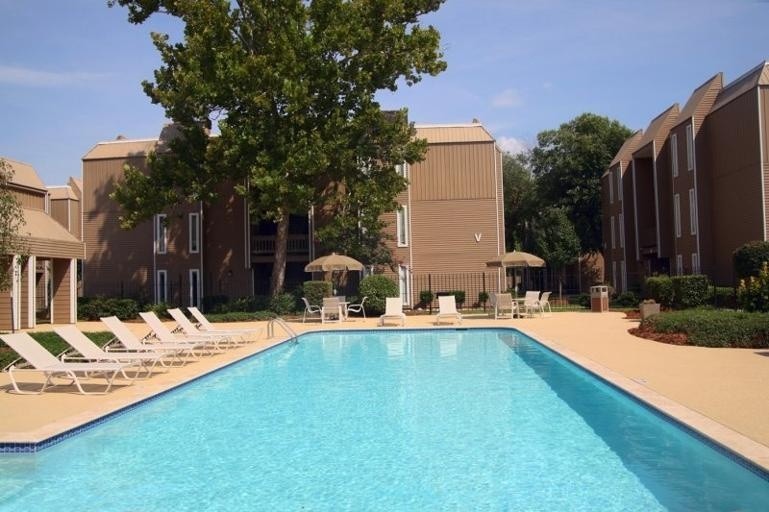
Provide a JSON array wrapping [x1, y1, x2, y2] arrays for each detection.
[[485, 249, 547, 299], [303, 253, 366, 295]]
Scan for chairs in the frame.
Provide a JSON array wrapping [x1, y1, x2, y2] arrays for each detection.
[[380, 297, 406, 327], [487, 290, 552, 322], [436, 294, 463, 326], [1, 305, 262, 397], [300, 294, 369, 324]]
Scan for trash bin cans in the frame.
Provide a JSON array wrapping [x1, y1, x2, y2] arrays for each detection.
[[590, 286, 608, 312]]
